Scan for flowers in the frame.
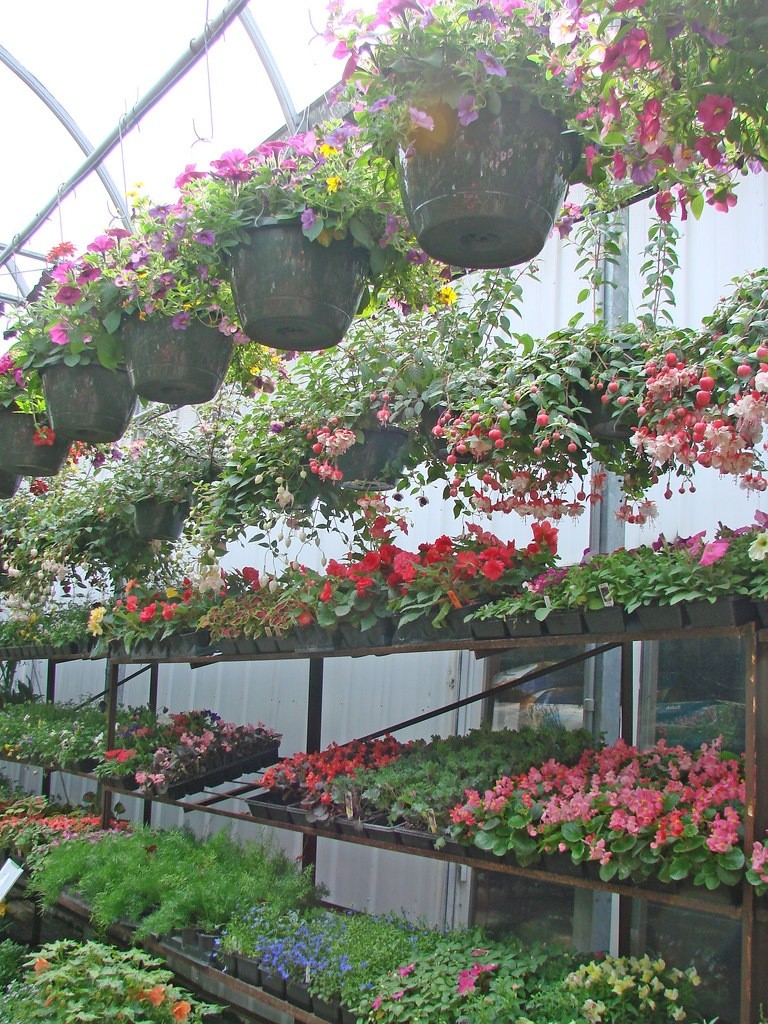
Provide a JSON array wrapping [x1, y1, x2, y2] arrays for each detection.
[[1, 4, 768, 457], [2, 266, 767, 598], [1, 937, 225, 1024], [1, 693, 405, 810], [0, 774, 135, 871], [1, 519, 768, 654], [212, 900, 738, 1024], [451, 732, 767, 896]]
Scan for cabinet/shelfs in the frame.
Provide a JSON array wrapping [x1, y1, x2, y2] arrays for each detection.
[[3, 323, 767, 1024]]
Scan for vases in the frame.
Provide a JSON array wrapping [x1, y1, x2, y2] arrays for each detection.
[[573, 368, 633, 440], [0, 847, 35, 875], [4, 66, 614, 499], [108, 736, 368, 841], [422, 399, 500, 462], [128, 493, 187, 539], [232, 953, 261, 986], [531, 843, 738, 909], [0, 584, 768, 665], [340, 1003, 358, 1024], [257, 966, 287, 1000], [327, 424, 405, 491], [213, 945, 237, 977], [311, 991, 342, 1024], [285, 977, 312, 1013]]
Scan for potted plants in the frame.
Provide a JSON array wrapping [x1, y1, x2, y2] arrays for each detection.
[[20, 808, 332, 969], [329, 723, 611, 863]]
